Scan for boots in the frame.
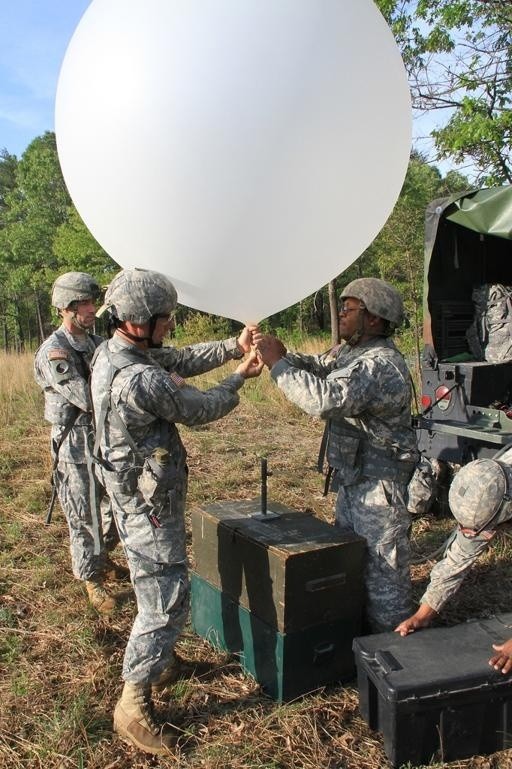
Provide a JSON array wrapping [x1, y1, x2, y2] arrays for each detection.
[[85, 554, 130, 612], [113, 684, 178, 755], [150, 650, 211, 688]]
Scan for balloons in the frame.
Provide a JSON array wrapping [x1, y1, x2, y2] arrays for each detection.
[[55, 0, 415, 332]]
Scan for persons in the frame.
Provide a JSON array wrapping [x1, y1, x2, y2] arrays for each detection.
[[88, 268, 264, 757], [390, 448, 512, 678], [31, 270, 128, 611], [252, 277, 419, 629]]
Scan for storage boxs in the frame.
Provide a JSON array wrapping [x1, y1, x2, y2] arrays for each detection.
[[351, 611, 512, 769], [193, 497, 368, 634], [183, 571, 372, 705]]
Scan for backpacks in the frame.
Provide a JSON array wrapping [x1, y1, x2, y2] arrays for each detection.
[[466, 284, 512, 363]]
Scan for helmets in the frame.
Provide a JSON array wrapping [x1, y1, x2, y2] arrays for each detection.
[[449, 459, 512, 530], [340, 278, 404, 326], [51, 271, 99, 309], [104, 268, 177, 325]]
